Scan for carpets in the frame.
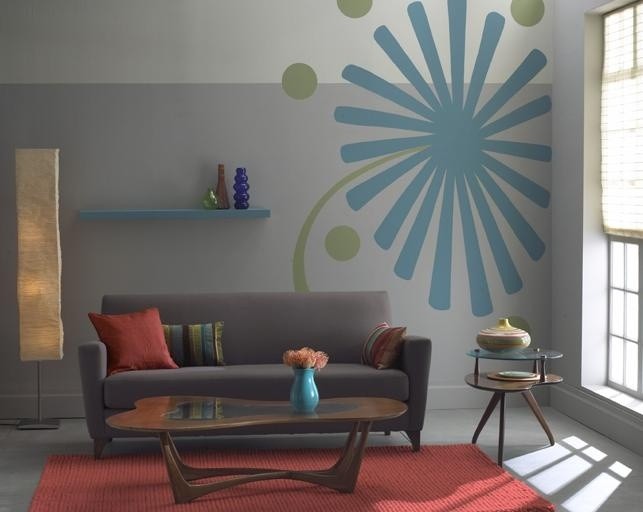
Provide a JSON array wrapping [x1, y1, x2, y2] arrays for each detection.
[[31, 443, 555, 512]]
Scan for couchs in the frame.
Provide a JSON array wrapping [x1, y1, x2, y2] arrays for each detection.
[[77, 292, 432, 460]]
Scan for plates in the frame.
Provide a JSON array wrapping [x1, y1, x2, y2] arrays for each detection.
[[498, 370, 536, 378]]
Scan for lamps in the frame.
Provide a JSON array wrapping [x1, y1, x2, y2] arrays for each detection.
[[15, 147, 64, 430]]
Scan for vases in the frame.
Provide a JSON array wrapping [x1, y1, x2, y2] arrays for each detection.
[[290, 368, 321, 416]]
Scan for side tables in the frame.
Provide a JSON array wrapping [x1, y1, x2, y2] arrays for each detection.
[[464, 348, 565, 469]]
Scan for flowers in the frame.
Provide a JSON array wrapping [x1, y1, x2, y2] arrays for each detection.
[[282, 346, 329, 370]]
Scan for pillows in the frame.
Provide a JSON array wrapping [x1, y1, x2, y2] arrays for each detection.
[[86, 306, 180, 375], [161, 320, 226, 366], [360, 321, 407, 369]]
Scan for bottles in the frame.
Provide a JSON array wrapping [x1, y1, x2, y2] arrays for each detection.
[[201, 187, 220, 210]]
